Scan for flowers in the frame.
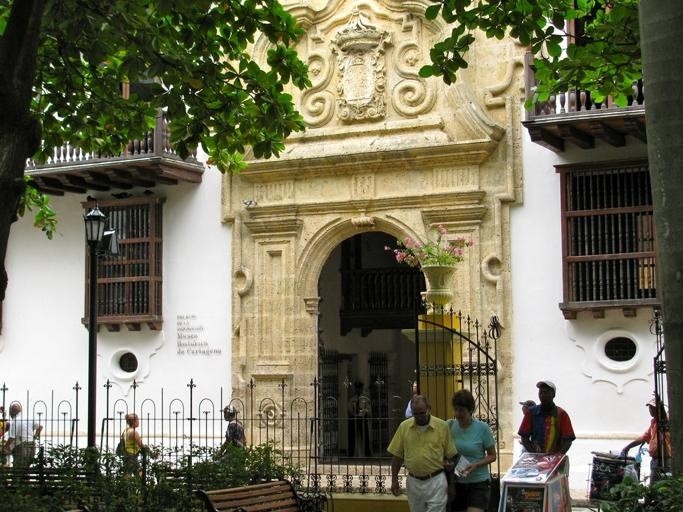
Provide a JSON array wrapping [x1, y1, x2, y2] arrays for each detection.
[[382, 222, 476, 268]]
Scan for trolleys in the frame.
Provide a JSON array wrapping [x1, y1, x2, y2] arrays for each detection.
[[584, 442, 647, 511], [498, 448, 574, 512]]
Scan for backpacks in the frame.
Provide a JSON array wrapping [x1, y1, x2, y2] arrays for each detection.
[[116, 429, 127, 459]]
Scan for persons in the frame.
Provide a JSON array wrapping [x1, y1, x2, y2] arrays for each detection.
[[518, 380, 575, 457], [388, 396, 454, 512], [519, 400, 537, 416], [440, 390, 497, 511], [405, 381, 419, 420], [622, 394, 672, 488], [118, 414, 159, 481], [212, 406, 246, 460], [0, 404, 43, 484]]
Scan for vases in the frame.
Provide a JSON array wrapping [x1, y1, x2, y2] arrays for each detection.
[[418, 263, 459, 314]]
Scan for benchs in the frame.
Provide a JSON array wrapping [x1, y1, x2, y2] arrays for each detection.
[[192, 476, 327, 512], [1, 466, 113, 507], [154, 467, 270, 497]]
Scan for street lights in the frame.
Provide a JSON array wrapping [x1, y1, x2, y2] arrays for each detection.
[[82, 201, 124, 477]]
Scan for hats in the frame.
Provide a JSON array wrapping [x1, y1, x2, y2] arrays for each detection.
[[645, 398, 662, 408], [536, 380, 556, 398], [224, 405, 238, 414], [518, 400, 536, 408]]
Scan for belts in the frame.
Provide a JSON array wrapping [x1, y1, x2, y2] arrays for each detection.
[[409, 469, 443, 481]]
